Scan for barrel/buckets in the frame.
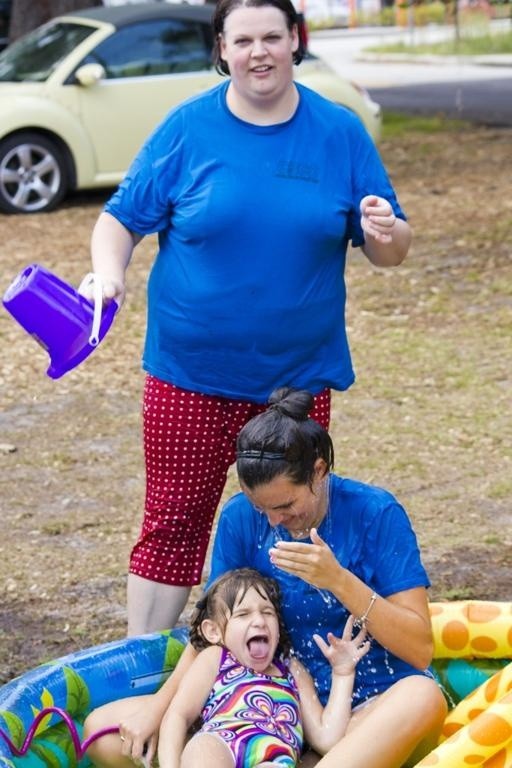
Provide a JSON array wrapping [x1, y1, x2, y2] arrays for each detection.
[[2, 263, 117, 378]]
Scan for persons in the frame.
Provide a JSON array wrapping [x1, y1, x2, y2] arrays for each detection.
[[77, 0, 416, 642], [154, 566, 371, 767], [80, 384, 451, 768]]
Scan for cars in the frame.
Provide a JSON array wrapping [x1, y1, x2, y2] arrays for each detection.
[[1, 3, 380, 217]]
